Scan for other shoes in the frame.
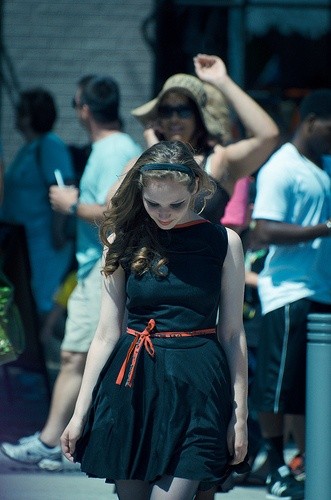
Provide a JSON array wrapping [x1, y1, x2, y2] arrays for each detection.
[[233, 473, 265, 486], [264, 452, 306, 500]]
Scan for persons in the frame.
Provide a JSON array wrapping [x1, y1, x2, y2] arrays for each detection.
[[108, 55, 278, 227], [205, 85, 244, 228], [61, 140, 247, 500], [236, 97, 307, 487], [0, 87, 82, 470], [3, 74, 145, 462], [252, 89, 331, 500]]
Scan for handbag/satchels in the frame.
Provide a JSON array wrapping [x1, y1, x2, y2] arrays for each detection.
[[0, 270, 25, 366], [55, 273, 78, 308]]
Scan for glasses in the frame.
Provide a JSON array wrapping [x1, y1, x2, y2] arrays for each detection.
[[158, 103, 198, 120], [70, 97, 84, 110]]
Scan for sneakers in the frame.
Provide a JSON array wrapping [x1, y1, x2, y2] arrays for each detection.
[[0, 432, 64, 472]]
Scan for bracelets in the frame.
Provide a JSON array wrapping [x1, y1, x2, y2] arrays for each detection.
[[69, 202, 79, 217]]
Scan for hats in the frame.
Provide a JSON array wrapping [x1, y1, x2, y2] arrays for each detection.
[[130, 74, 229, 146]]
